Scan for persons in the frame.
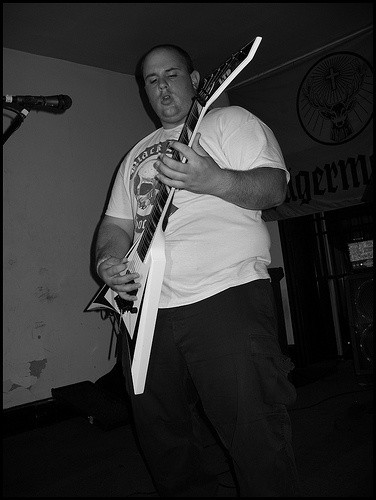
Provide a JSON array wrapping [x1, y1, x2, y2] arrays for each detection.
[[95, 44, 302, 500]]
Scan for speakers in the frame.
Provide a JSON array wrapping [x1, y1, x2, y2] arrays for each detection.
[[344, 273, 376, 383]]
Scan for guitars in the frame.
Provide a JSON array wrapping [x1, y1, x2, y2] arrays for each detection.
[[84, 34, 263, 398]]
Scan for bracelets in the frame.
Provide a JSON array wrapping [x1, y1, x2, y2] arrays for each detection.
[[96, 255, 123, 279]]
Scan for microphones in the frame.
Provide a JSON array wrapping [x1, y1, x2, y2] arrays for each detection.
[[3, 95, 72, 110]]
